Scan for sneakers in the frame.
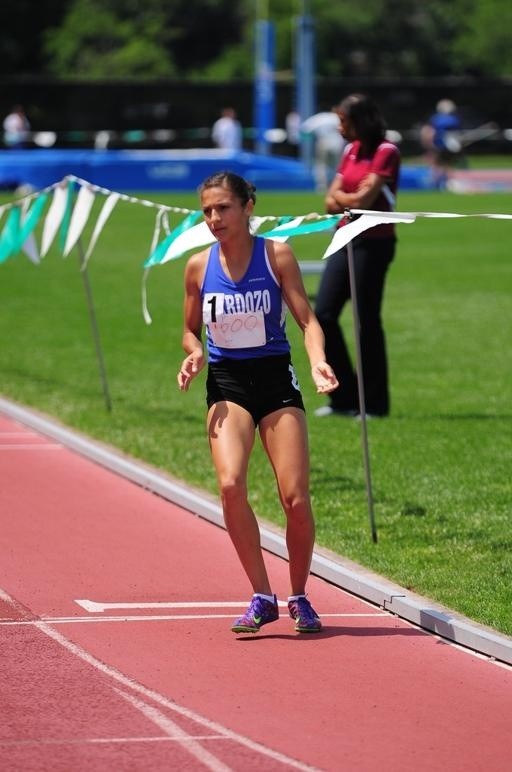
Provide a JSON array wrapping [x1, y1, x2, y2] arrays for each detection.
[[287, 592, 322, 633], [232, 593, 279, 632]]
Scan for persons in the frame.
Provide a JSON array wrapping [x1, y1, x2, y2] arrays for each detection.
[[300, 99, 349, 196], [174, 172, 344, 635], [210, 103, 245, 151], [418, 97, 471, 172], [2, 101, 36, 153], [309, 92, 402, 423]]
